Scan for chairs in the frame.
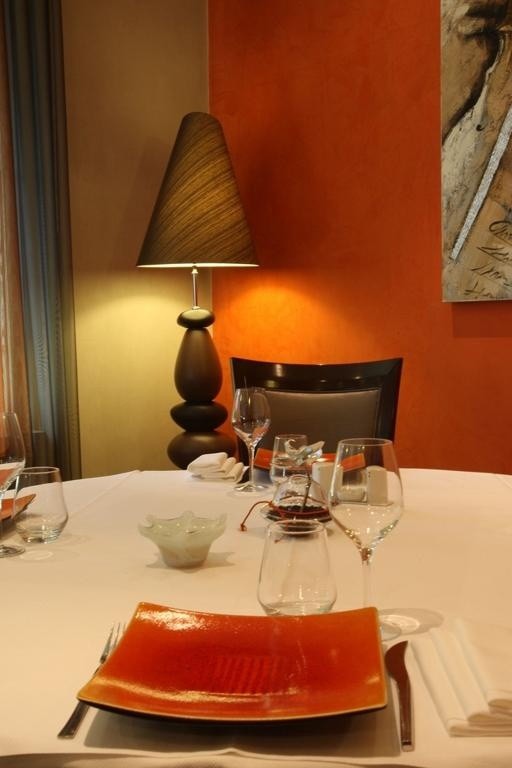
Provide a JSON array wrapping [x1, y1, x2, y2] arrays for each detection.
[[229, 358, 404, 476]]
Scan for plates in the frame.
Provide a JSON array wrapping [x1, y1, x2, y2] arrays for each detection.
[[253, 447, 366, 474], [0, 493, 36, 525], [76, 601, 388, 728]]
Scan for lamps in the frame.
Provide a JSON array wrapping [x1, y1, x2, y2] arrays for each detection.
[[137, 111, 256, 472]]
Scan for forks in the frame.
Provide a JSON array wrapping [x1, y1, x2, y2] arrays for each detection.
[[57, 621, 127, 740]]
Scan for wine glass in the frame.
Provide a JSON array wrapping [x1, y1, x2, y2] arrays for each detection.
[[0, 412, 26, 558], [328, 438, 405, 642], [230, 387, 272, 493]]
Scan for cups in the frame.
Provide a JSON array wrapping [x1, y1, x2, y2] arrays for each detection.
[[14, 466, 68, 543], [268, 432, 314, 486], [312, 462, 343, 504], [257, 519, 337, 616]]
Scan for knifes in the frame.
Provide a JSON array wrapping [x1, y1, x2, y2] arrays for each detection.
[[384, 641, 413, 745]]
